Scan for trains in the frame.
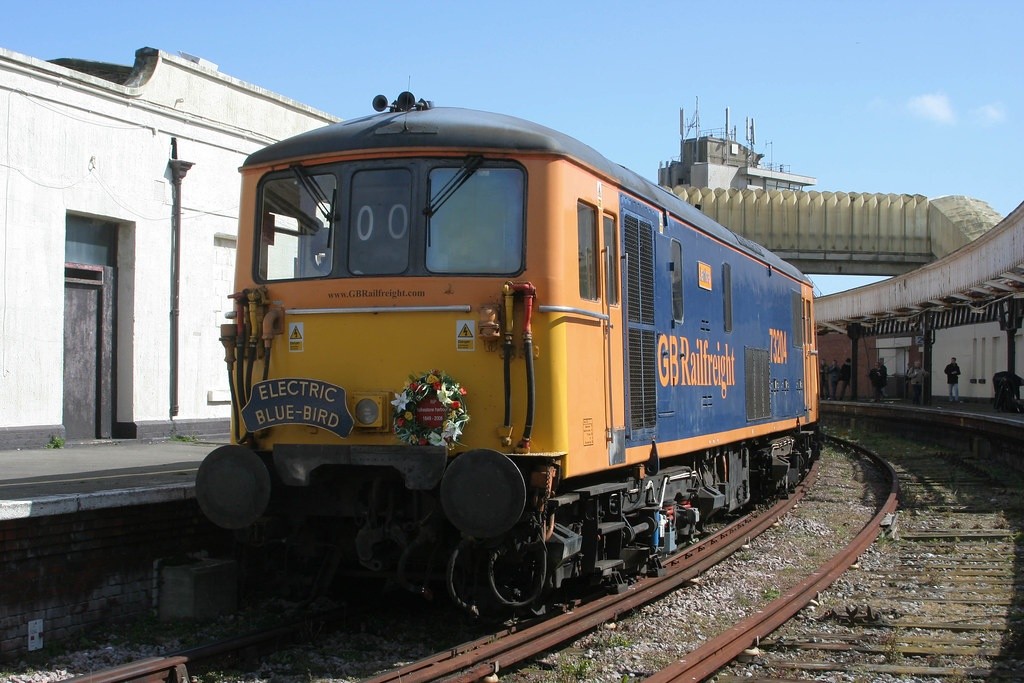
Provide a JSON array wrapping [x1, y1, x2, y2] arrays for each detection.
[[194, 91, 822, 620]]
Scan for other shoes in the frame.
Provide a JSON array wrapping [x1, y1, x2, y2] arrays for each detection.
[[913, 400, 921, 406], [874, 399, 881, 403], [955, 399, 959, 403], [949, 399, 954, 404], [827, 397, 832, 401]]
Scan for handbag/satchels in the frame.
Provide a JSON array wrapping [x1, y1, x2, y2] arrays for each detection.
[[904, 367, 914, 384]]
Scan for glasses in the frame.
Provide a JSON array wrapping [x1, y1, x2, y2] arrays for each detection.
[[874, 365, 878, 366]]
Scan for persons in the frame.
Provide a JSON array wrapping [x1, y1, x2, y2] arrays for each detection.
[[869, 358, 888, 403], [908, 361, 925, 407], [839, 358, 851, 401], [819, 359, 831, 400], [944, 357, 961, 404], [828, 360, 840, 401]]
